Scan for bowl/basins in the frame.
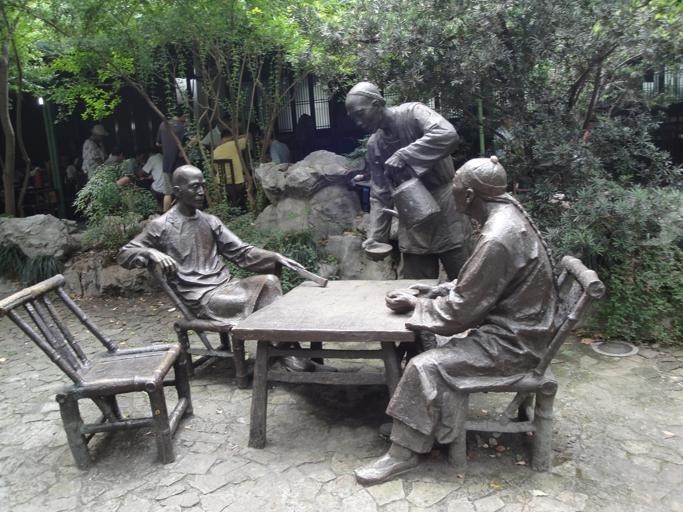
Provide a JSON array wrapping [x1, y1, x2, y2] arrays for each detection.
[[392, 287, 420, 297], [365, 242, 394, 260]]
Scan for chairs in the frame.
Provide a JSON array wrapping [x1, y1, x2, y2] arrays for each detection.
[[448, 255, 606, 473], [134, 250, 256, 389], [209, 158, 248, 214], [0, 272, 194, 471]]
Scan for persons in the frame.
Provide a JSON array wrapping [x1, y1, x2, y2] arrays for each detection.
[[344, 81, 470, 368], [113, 162, 340, 375], [82, 125, 110, 179], [353, 154, 557, 486], [289, 112, 316, 162], [125, 106, 289, 215], [13, 153, 89, 215]]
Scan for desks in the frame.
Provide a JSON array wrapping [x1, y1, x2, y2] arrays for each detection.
[[231, 278, 443, 450]]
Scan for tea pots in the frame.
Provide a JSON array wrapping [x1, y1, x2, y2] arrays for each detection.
[[380, 165, 443, 231]]
